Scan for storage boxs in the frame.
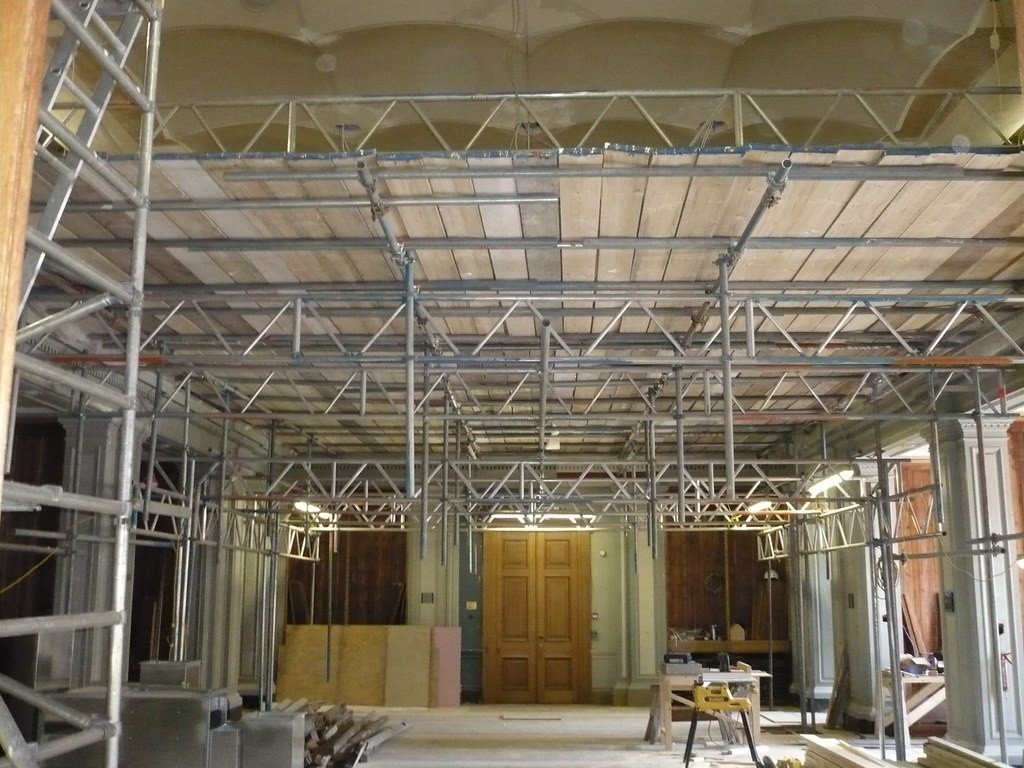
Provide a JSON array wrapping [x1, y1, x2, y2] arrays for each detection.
[[140, 660, 202, 692], [900, 658, 932, 674]]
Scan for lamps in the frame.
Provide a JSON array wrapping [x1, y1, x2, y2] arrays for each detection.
[[806, 466, 854, 496]]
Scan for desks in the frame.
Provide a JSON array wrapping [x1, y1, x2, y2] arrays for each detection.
[[643, 665, 771, 750]]
